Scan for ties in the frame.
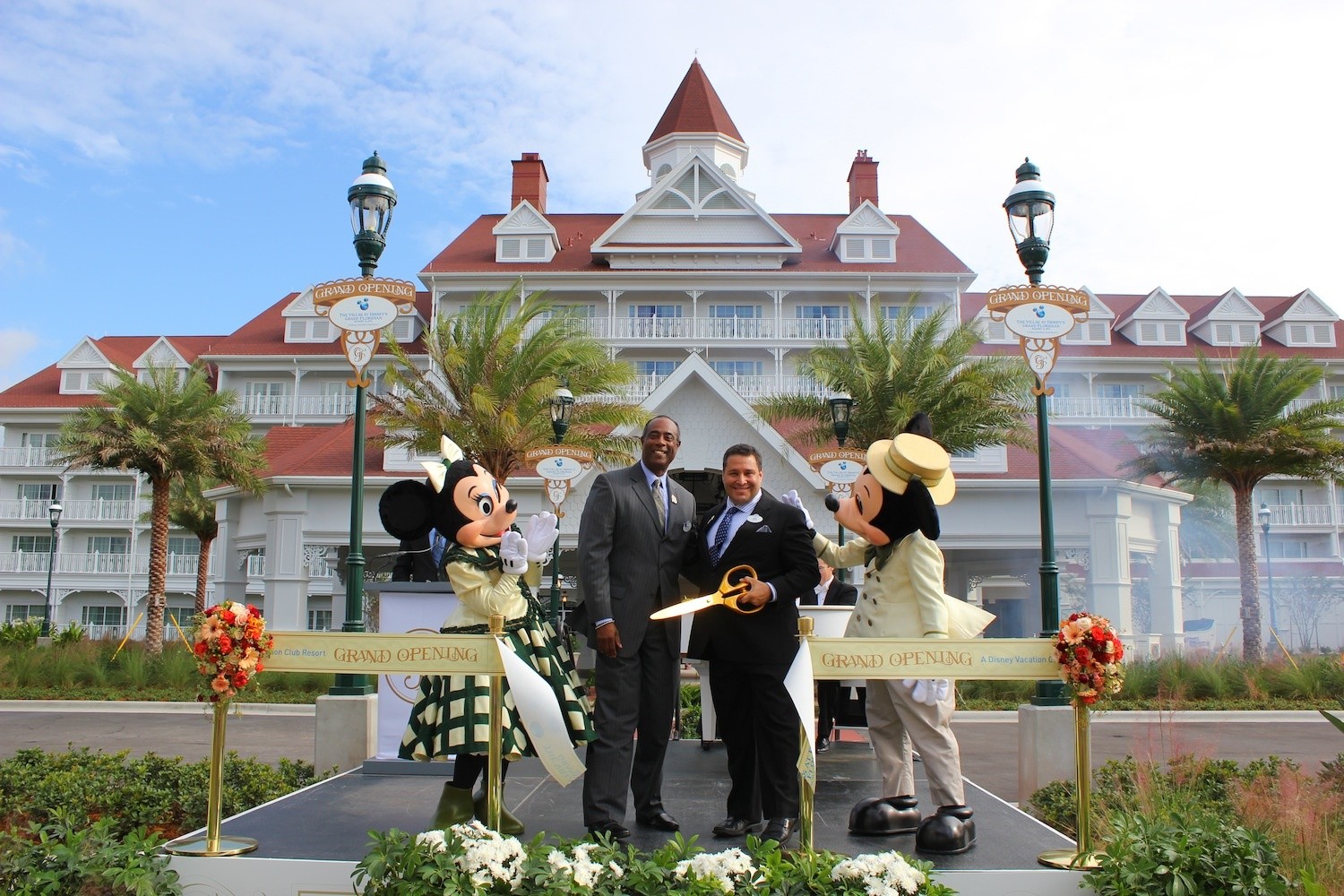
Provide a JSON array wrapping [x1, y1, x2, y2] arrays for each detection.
[[711, 506, 739, 569], [651, 479, 666, 535]]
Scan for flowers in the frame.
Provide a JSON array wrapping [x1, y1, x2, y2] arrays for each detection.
[[183, 595, 275, 716], [1046, 613, 1126, 707]]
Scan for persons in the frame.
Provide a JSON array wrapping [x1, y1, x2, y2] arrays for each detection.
[[799, 557, 858, 751], [563, 416, 702, 837], [686, 444, 821, 848]]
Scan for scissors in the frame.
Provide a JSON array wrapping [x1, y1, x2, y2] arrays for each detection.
[[650, 564, 768, 619]]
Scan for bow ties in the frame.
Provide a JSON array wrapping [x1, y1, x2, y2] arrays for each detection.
[[814, 584, 827, 594]]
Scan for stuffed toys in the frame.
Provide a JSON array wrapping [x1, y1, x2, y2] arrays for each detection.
[[781, 433, 997, 853], [379, 435, 599, 836]]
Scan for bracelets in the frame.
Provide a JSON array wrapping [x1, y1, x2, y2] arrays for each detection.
[[595, 620, 614, 629]]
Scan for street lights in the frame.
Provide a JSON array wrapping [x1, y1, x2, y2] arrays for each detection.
[[36, 499, 64, 653], [1002, 156, 1082, 807], [1257, 502, 1284, 658], [827, 384, 858, 584], [541, 388, 575, 635], [315, 148, 400, 778]]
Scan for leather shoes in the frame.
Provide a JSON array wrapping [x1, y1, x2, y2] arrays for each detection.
[[591, 825, 631, 840], [635, 810, 680, 831], [815, 737, 829, 753], [712, 816, 751, 836], [759, 815, 799, 848]]
